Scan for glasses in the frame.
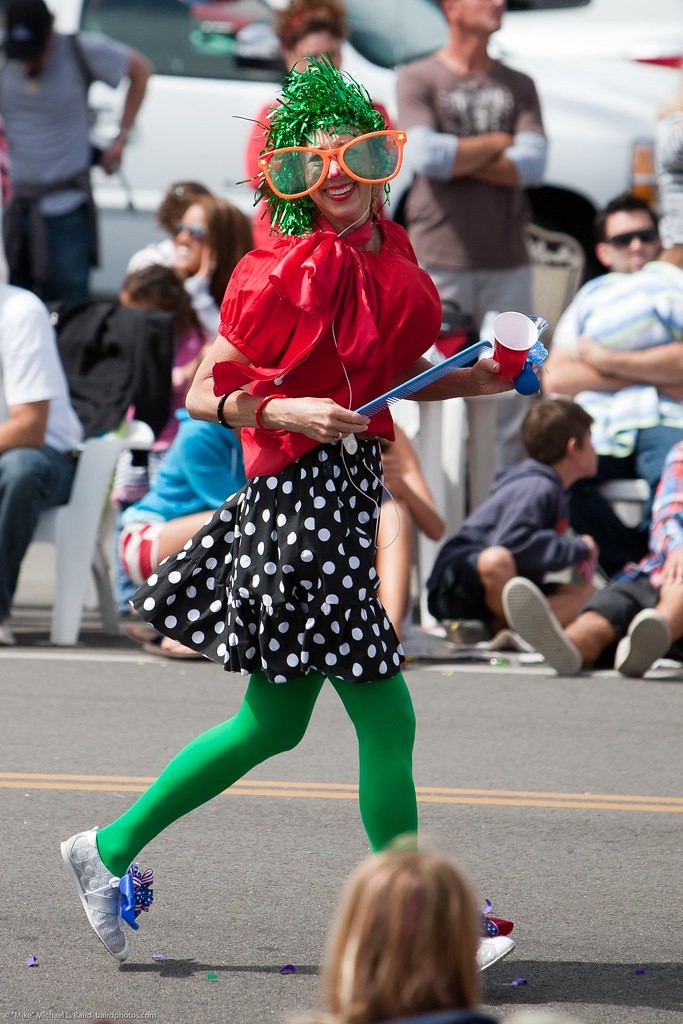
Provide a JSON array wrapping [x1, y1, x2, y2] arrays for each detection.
[[175, 222, 207, 240], [610, 228, 659, 247], [259, 130, 408, 199]]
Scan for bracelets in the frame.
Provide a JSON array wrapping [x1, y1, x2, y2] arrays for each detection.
[[254, 394, 290, 437], [216, 388, 244, 429], [116, 130, 127, 142]]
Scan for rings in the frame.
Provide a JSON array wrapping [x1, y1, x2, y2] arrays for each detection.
[[334, 431, 342, 441]]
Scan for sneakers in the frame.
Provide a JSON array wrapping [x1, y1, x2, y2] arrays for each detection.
[[501, 577, 583, 676], [475, 934, 515, 975], [60, 827, 130, 963], [614, 608, 670, 677]]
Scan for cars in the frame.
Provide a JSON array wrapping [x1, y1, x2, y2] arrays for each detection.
[[0, 0, 683, 289]]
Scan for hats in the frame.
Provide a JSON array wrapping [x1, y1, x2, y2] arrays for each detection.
[[1, 0, 53, 59]]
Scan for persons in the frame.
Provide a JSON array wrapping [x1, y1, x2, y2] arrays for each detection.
[[0, 0, 683, 679], [319, 846, 502, 1024], [59, 53, 542, 976]]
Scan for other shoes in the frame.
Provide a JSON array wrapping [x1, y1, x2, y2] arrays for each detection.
[[143, 634, 205, 659], [122, 619, 159, 644], [642, 657, 682, 678]]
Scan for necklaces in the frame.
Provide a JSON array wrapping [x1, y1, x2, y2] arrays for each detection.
[[370, 225, 379, 251]]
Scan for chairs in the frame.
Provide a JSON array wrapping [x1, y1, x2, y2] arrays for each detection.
[[30, 421, 155, 646]]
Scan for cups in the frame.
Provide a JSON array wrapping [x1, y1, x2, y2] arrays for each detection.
[[490, 310, 539, 383]]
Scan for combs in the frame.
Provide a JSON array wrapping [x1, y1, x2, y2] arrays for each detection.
[[354, 340, 493, 418]]
